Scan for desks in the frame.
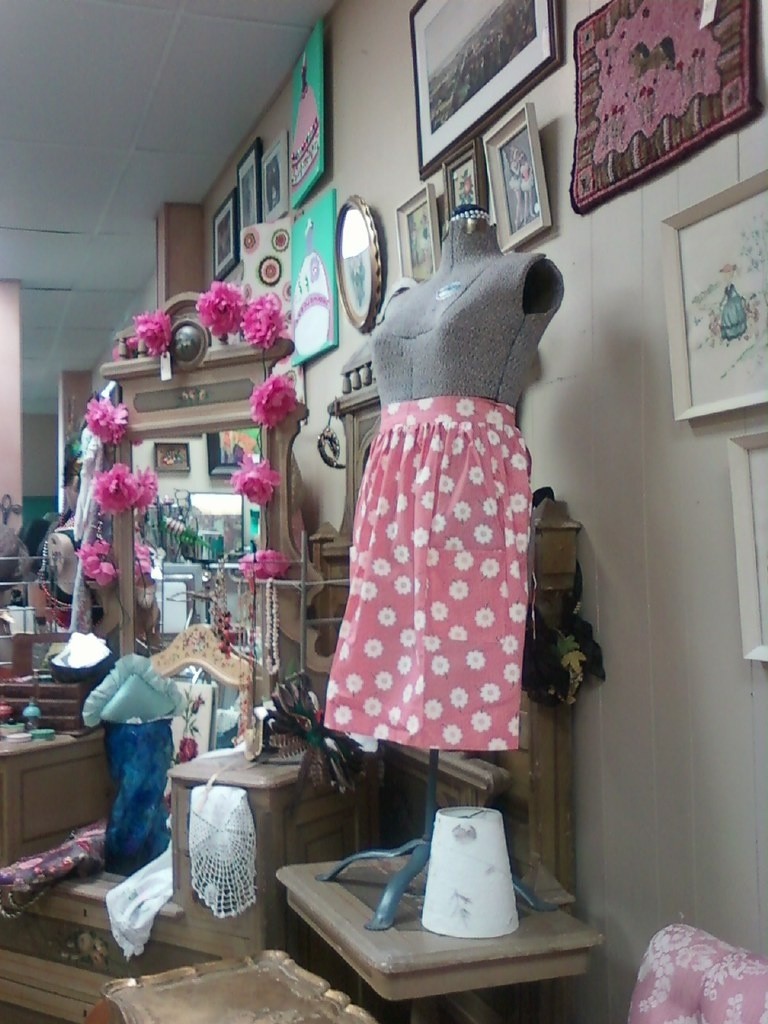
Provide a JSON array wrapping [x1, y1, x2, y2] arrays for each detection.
[[278, 860, 600, 1019]]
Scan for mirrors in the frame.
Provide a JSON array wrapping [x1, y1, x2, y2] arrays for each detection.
[[187, 491, 245, 563], [99, 288, 294, 759]]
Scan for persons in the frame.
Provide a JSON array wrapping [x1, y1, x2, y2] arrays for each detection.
[[325, 210, 565, 754]]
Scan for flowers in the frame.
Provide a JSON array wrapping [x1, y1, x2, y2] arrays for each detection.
[[87, 399, 130, 448], [254, 546, 290, 579], [76, 540, 118, 584], [241, 554, 257, 579], [134, 307, 171, 357], [232, 456, 281, 506], [239, 294, 287, 350], [130, 467, 161, 509], [132, 542, 155, 589], [196, 281, 243, 338], [250, 375, 298, 429], [92, 462, 142, 516]]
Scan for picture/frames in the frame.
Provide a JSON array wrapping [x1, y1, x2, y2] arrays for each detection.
[[441, 138, 488, 218], [410, 0, 564, 180], [484, 102, 552, 254], [727, 428, 768, 663], [213, 186, 237, 283], [395, 183, 442, 281], [663, 169, 767, 421], [336, 194, 383, 334], [207, 426, 261, 478], [262, 129, 288, 222], [152, 441, 190, 471], [236, 137, 261, 259]]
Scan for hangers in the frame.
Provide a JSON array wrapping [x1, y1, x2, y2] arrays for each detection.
[[146, 592, 254, 689]]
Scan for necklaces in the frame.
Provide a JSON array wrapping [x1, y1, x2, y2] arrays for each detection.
[[238, 570, 255, 737], [41, 541, 73, 611], [263, 577, 281, 676], [208, 563, 234, 662]]
